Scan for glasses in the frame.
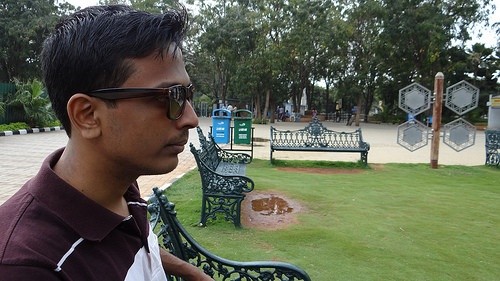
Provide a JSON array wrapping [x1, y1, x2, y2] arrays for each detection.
[[86, 84, 195, 120]]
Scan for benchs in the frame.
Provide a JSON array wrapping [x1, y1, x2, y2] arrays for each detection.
[[189, 126, 254, 227], [270, 115, 370, 165], [146, 187, 310, 281]]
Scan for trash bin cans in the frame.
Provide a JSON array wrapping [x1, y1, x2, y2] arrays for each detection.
[[233, 109, 252, 144], [212, 109, 231, 144]]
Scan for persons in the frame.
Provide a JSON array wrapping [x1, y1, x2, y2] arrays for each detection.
[[222, 104, 237, 116], [275, 105, 301, 122], [313, 108, 317, 117], [0, 3, 216, 281], [336, 110, 340, 122]]
